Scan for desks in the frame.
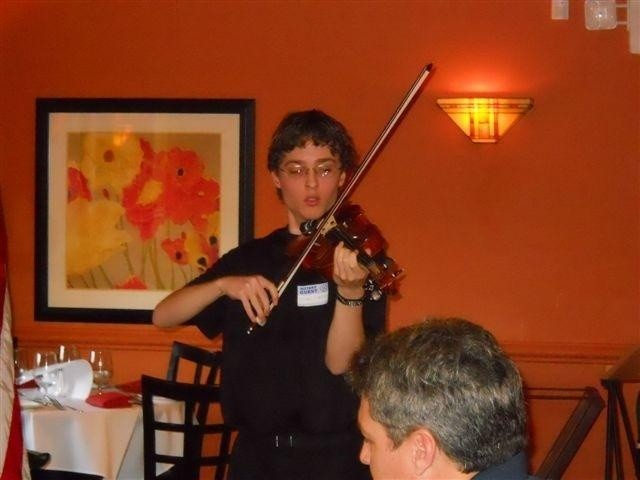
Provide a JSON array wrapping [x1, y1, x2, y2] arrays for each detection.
[[15, 378, 192, 479]]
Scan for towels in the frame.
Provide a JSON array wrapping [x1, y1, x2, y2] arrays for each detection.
[[85, 390, 132, 409], [117, 376, 141, 393]]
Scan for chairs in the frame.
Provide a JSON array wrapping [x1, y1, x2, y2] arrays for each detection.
[[161, 343, 227, 477], [136, 376, 236, 479]]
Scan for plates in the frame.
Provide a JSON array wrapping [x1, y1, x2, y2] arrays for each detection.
[[20, 393, 47, 408]]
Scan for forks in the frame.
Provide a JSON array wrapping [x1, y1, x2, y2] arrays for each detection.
[[18, 391, 45, 405], [47, 395, 69, 410]]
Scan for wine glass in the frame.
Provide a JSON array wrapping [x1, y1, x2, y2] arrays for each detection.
[[30, 349, 59, 407], [59, 343, 85, 362], [12, 347, 26, 379], [88, 348, 114, 396]]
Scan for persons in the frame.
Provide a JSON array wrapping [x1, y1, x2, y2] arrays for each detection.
[[346, 314, 541, 478], [152, 109, 388, 480]]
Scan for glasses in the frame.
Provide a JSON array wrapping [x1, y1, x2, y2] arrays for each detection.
[[280, 166, 341, 178]]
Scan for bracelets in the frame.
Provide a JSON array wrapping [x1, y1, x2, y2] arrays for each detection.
[[336, 290, 368, 307]]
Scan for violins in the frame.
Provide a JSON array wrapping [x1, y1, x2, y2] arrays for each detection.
[[284, 203, 406, 303]]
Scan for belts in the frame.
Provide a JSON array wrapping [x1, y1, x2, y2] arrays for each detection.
[[262, 434, 327, 449]]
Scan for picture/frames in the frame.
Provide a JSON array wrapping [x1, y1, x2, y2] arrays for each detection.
[[34, 95, 257, 329]]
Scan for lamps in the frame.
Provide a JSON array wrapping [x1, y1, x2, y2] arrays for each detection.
[[435, 93, 535, 145]]
